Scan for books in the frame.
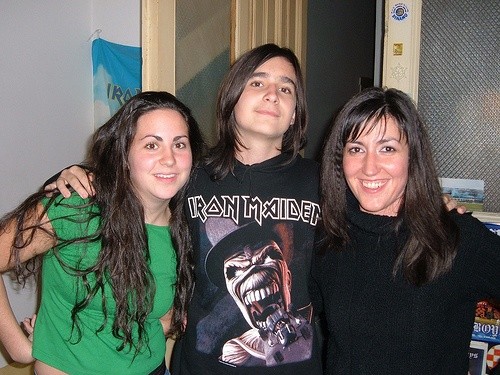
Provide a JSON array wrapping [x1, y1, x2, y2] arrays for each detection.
[[469, 222, 500, 344], [467, 339, 489, 375]]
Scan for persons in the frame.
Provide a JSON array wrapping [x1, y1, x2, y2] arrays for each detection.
[[306, 85, 500, 375], [43, 42, 472, 375], [1, 90, 212, 375]]
